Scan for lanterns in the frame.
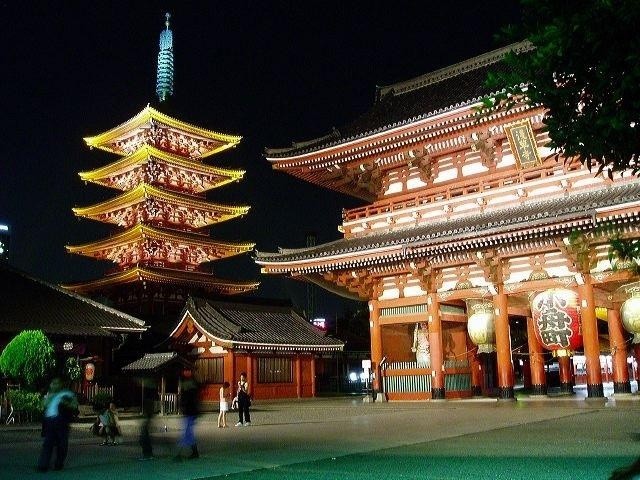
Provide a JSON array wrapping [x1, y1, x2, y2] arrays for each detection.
[[530, 286, 583, 358], [619, 280, 640, 343], [85, 363, 95, 381], [464, 298, 505, 354]]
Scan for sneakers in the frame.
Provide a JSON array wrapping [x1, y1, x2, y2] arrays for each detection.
[[235, 422, 252, 427], [217, 424, 229, 428], [99, 441, 118, 446]]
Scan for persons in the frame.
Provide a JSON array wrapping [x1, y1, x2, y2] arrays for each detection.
[[35, 377, 78, 472], [411, 322, 430, 362], [138, 373, 157, 461], [173, 364, 201, 462], [90, 401, 123, 446], [235, 372, 251, 427], [218, 382, 231, 428]]
